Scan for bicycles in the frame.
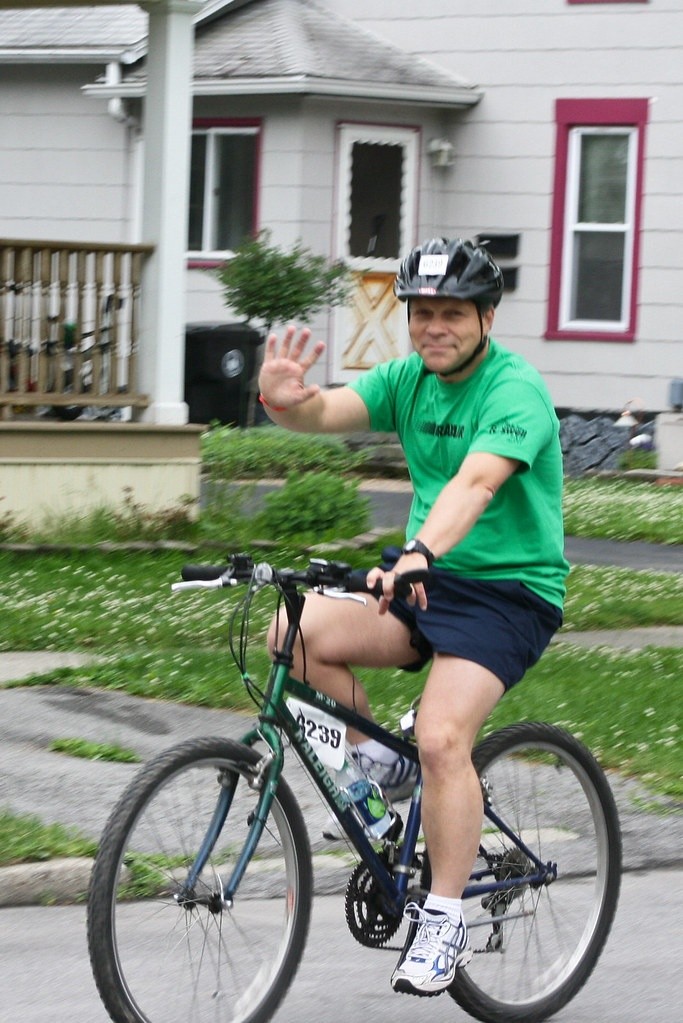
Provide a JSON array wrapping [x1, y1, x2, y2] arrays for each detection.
[[76, 548, 624, 1022], [32, 296, 123, 419]]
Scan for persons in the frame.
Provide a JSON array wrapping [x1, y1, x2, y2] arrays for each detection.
[[257, 234, 573, 999]]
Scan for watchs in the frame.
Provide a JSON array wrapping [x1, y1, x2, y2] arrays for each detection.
[[400, 538, 435, 569]]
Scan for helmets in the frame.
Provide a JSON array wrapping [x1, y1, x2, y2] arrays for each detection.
[[394, 240, 507, 305]]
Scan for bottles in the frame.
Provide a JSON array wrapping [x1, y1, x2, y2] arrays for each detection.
[[329, 757, 393, 840]]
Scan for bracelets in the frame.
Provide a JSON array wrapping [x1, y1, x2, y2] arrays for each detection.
[[258, 394, 288, 412]]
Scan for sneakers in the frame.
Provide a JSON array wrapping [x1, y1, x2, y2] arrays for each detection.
[[387, 907, 472, 997], [322, 749, 418, 843]]
